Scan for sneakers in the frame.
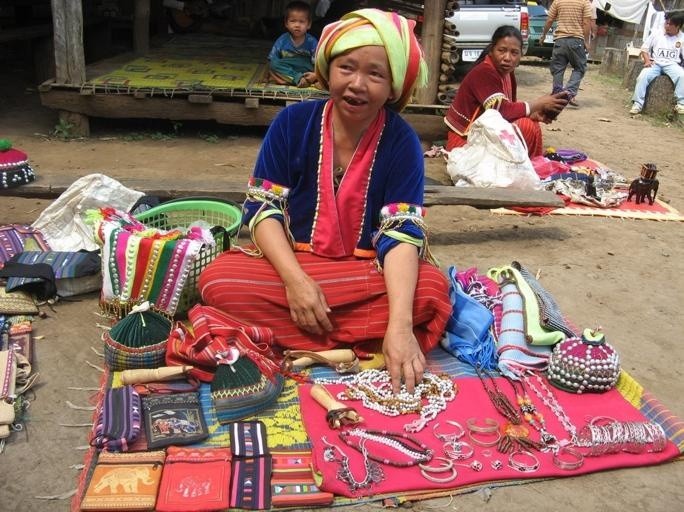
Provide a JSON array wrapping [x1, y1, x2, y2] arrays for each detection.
[[569, 96, 578, 106], [674, 105, 684, 114], [629, 105, 641, 114]]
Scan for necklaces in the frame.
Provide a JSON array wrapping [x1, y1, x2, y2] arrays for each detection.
[[318, 371, 459, 434], [502, 365, 567, 450], [475, 364, 538, 453], [323, 427, 436, 493], [518, 374, 579, 442]]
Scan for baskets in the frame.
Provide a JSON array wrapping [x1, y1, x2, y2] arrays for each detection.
[[105, 195, 243, 320]]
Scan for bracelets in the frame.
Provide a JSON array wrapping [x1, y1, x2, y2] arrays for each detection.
[[416, 414, 502, 483], [509, 450, 540, 472], [577, 416, 669, 454], [550, 446, 583, 469]]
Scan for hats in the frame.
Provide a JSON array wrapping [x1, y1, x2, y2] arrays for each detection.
[[0, 139, 35, 189], [546, 326, 620, 394], [211, 348, 285, 424], [103, 301, 172, 373]]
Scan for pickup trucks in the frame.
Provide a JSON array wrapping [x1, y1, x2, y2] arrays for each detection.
[[444, 0, 530, 70]]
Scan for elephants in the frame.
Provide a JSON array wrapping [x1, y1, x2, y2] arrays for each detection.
[[626, 177, 660, 206]]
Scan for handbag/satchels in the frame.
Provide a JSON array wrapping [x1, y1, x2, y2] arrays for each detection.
[[0, 293, 51, 441], [0, 171, 161, 299], [441, 109, 545, 190], [268, 447, 333, 506], [77, 447, 166, 510], [142, 390, 209, 448], [95, 385, 141, 454], [155, 446, 231, 511]]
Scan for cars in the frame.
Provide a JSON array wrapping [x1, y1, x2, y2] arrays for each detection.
[[524, 0, 562, 59]]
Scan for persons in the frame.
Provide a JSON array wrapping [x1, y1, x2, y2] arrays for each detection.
[[626, 12, 683, 116], [443, 28, 570, 186], [538, 1, 593, 109], [202, 8, 450, 395], [258, 2, 324, 90], [575, 7, 597, 91]]
